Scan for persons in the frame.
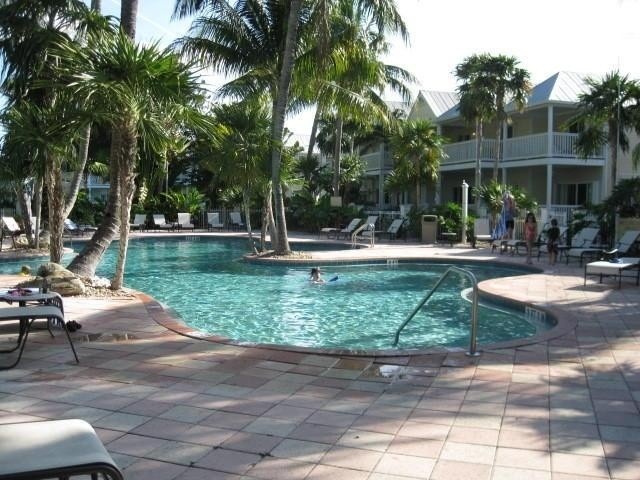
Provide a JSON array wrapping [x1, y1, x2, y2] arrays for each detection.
[[307, 267, 325, 285], [545, 218, 560, 265], [521, 213, 538, 265], [502, 193, 518, 254]]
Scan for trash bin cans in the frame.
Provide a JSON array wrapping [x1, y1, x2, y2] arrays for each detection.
[[421, 214, 438, 242]]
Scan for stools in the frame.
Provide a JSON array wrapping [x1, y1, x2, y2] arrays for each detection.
[[473, 235, 492, 249], [490, 240, 508, 255], [0, 419, 125, 479]]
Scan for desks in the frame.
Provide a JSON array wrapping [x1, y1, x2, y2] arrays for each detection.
[[566, 248, 605, 267], [0, 290, 57, 337], [584, 257, 640, 289]]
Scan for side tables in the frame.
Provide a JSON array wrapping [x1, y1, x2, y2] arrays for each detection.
[[589, 244, 609, 262]]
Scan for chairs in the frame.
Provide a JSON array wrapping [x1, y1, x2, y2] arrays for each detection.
[[0, 306, 79, 370], [153, 214, 172, 233], [130, 213, 146, 233], [229, 213, 247, 232], [506, 226, 570, 257], [1, 213, 98, 251], [439, 216, 457, 247], [178, 213, 195, 232], [589, 231, 640, 268], [321, 214, 404, 243], [206, 213, 224, 233], [536, 228, 601, 262]]
